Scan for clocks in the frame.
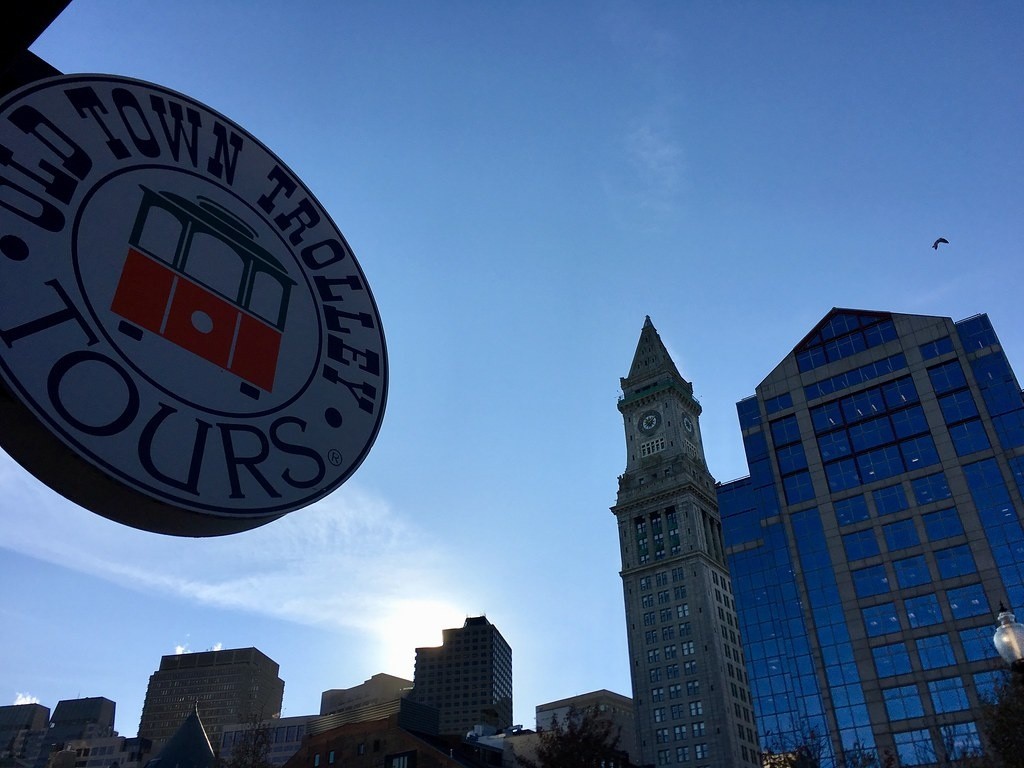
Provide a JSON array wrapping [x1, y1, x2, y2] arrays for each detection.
[[682, 412, 694, 437], [638, 410, 662, 436]]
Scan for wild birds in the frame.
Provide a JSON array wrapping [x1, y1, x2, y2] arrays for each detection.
[[932, 237, 948, 249]]
